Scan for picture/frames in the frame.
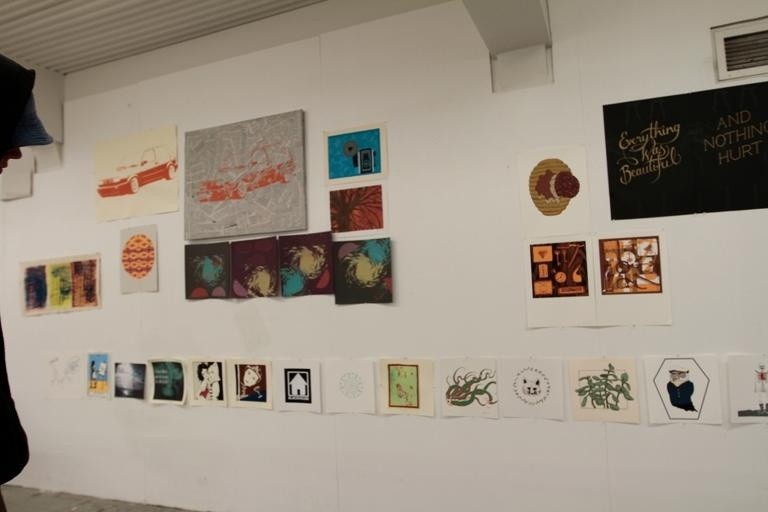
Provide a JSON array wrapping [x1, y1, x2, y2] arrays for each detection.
[[322, 122, 388, 186]]
